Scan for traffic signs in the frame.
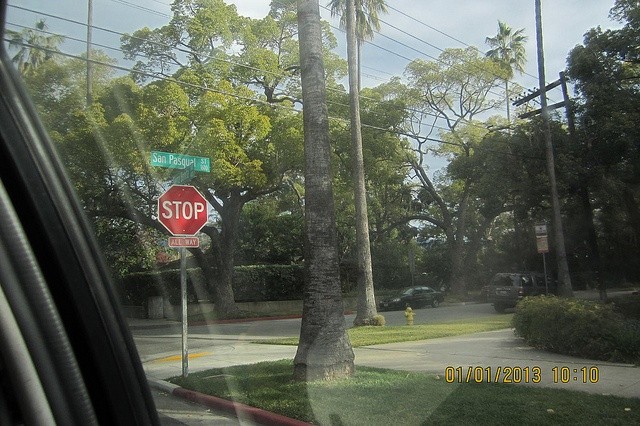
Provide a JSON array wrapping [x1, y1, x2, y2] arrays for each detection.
[[168, 236, 200, 248], [171, 163, 198, 186], [150, 151, 211, 173]]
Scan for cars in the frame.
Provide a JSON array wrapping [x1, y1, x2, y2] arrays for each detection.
[[379, 286, 444, 311]]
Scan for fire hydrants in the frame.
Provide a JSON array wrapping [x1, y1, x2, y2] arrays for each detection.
[[405, 307, 416, 326]]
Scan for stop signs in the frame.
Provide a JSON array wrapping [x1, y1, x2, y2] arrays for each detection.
[[157, 185, 208, 236]]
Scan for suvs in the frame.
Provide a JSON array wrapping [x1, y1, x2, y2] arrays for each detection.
[[487, 271, 556, 312]]
